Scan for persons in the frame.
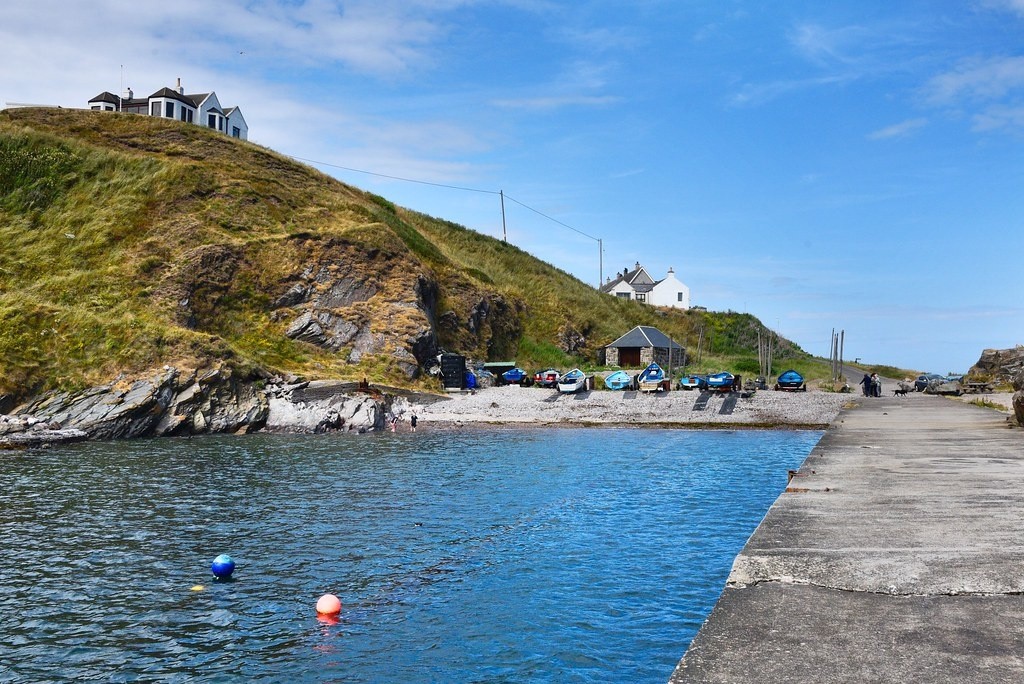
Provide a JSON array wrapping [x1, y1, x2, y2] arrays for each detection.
[[389, 415, 399, 433], [857, 371, 884, 399], [410, 412, 418, 432]]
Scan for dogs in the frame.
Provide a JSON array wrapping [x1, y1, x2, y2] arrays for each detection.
[[890, 389, 907, 398]]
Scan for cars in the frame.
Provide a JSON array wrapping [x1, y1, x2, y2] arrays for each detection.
[[946, 375, 964, 384], [914, 373, 949, 393]]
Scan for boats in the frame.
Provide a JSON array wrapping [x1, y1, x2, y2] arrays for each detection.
[[637, 362, 665, 392], [534, 367, 561, 387], [777, 369, 803, 387], [558, 368, 586, 392], [704, 370, 736, 391], [501, 367, 528, 383], [604, 370, 631, 390], [681, 375, 707, 390]]
